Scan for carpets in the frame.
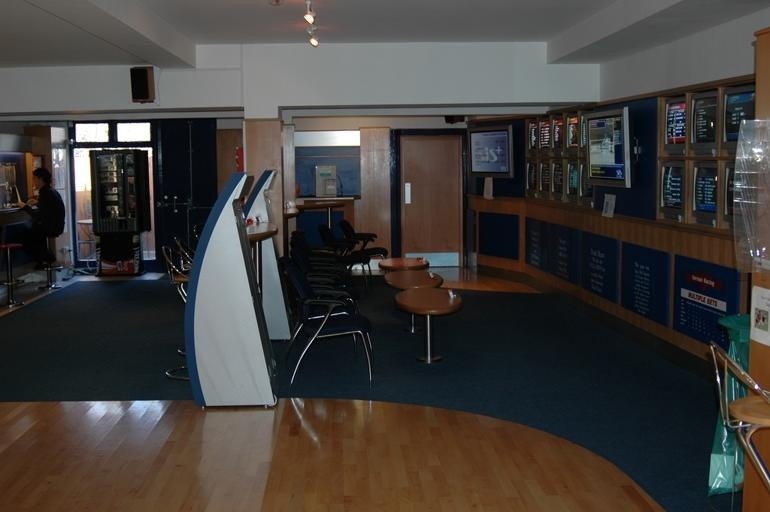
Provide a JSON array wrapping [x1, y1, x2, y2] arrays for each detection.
[[1, 275, 742, 512]]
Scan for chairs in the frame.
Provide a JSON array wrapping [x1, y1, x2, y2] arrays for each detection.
[[286, 219, 388, 391], [162, 223, 196, 380]]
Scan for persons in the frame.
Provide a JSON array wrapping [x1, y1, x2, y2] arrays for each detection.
[[6, 167, 66, 274]]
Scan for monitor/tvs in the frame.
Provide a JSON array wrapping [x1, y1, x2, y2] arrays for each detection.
[[691, 88, 722, 158], [656, 158, 689, 225], [657, 92, 690, 156], [468, 125, 513, 178], [586, 107, 632, 188], [690, 160, 725, 225], [722, 161, 735, 229], [723, 87, 754, 156], [526, 115, 595, 202]]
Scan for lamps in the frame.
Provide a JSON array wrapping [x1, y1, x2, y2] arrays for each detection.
[[303, 0, 320, 47]]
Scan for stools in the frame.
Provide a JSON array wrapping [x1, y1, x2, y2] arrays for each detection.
[[394, 287, 463, 365], [379, 258, 429, 270], [0, 243, 25, 309], [385, 269, 443, 334], [38, 235, 63, 290]]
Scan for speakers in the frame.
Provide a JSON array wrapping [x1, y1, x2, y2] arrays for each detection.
[[130, 66, 155, 102]]
